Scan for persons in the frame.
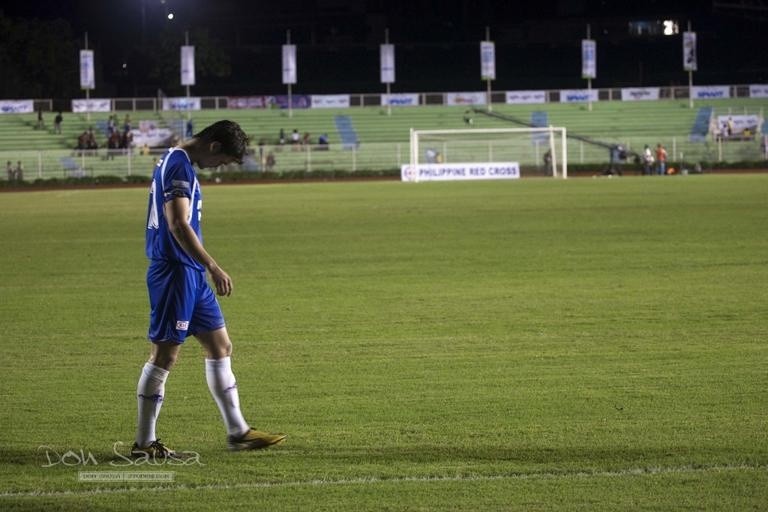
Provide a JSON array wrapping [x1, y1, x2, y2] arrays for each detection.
[[5, 160, 15, 180], [130, 119, 290, 460], [603, 140, 704, 175], [34, 106, 150, 157], [207, 124, 332, 173], [543, 148, 553, 176], [186, 119, 193, 136], [13, 161, 23, 179], [708, 116, 754, 141]]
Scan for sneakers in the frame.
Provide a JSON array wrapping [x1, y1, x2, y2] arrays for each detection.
[[226, 427, 288, 450], [131, 441, 184, 459]]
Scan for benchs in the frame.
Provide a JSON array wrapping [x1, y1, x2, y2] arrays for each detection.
[[0, 98, 768, 182]]
